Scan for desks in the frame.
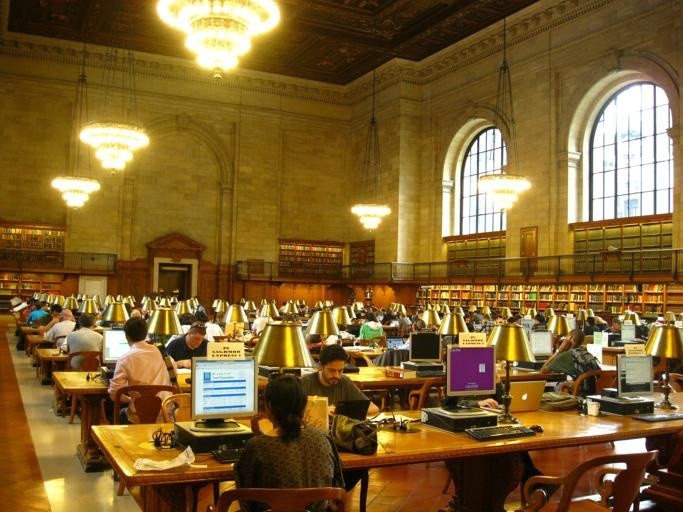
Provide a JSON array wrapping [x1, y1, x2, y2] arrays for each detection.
[[53, 357, 619, 472], [89, 392, 682, 512], [22, 326, 44, 349], [35, 348, 69, 385], [383, 326, 397, 337], [27, 335, 43, 356], [603, 347, 625, 366]]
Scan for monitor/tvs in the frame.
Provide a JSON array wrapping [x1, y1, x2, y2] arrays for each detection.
[[192, 356, 258, 432], [439, 344, 497, 415], [528, 331, 553, 357], [620, 324, 636, 341], [617, 357, 660, 402], [103, 329, 132, 371], [409, 331, 442, 362]]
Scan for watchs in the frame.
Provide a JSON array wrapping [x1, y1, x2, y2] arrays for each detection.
[[556, 349, 560, 353]]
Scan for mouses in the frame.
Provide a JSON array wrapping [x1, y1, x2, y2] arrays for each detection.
[[670, 405, 677, 410], [530, 420, 544, 437]]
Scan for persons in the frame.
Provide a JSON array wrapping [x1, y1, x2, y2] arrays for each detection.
[[378, 308, 387, 318], [594, 316, 608, 331], [32, 304, 63, 367], [41, 309, 78, 385], [130, 303, 143, 319], [278, 302, 288, 317], [582, 317, 600, 335], [532, 313, 548, 331], [163, 322, 210, 369], [396, 312, 411, 325], [61, 319, 87, 354], [194, 304, 206, 321], [571, 319, 603, 347], [107, 317, 172, 427], [541, 329, 606, 396], [236, 375, 346, 512], [381, 307, 397, 325], [23, 300, 49, 330], [393, 319, 427, 350], [615, 309, 641, 344], [507, 316, 516, 325], [190, 311, 224, 344], [480, 314, 496, 339], [525, 310, 535, 320], [515, 314, 523, 325], [412, 311, 423, 324], [378, 306, 386, 313], [65, 312, 107, 376], [76, 298, 84, 309], [37, 304, 83, 351], [474, 310, 485, 324], [463, 314, 475, 332], [371, 303, 377, 309], [360, 312, 383, 347], [37, 302, 50, 315], [361, 310, 368, 319], [23, 298, 35, 315], [21, 301, 37, 322], [301, 345, 379, 492], [365, 307, 376, 318], [164, 313, 213, 346], [251, 306, 276, 336], [606, 318, 613, 333], [467, 311, 476, 323], [493, 313, 504, 326]]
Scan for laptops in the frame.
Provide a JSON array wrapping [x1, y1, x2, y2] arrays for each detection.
[[480, 380, 545, 414], [336, 400, 370, 421], [389, 319, 400, 327], [387, 337, 403, 349]]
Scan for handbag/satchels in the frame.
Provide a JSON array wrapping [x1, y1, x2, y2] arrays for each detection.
[[332, 415, 378, 456]]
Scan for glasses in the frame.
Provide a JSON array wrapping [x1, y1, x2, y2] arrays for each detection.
[[189, 324, 209, 329]]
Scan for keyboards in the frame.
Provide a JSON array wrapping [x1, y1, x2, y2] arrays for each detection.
[[465, 425, 535, 441], [630, 412, 683, 422], [415, 370, 445, 377], [213, 448, 247, 463]]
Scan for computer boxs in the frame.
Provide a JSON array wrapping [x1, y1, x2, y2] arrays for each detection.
[[403, 362, 443, 373], [421, 406, 497, 433], [615, 340, 639, 346], [585, 394, 659, 416], [174, 421, 252, 454], [100, 367, 121, 385]]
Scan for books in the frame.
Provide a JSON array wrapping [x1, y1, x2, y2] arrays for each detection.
[[0, 227, 64, 264], [416, 283, 664, 319]]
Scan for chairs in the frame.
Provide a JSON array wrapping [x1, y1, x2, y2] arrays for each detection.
[[114, 385, 182, 424], [516, 451, 658, 512], [560, 370, 618, 397], [669, 374, 682, 392], [162, 394, 192, 422], [361, 336, 386, 347], [408, 377, 447, 410], [206, 488, 352, 512], [55, 335, 67, 347], [64, 351, 103, 373]]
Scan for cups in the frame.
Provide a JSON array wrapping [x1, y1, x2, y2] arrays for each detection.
[[587, 402, 600, 416]]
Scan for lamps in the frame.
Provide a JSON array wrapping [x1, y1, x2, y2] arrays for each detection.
[[159, 298, 170, 307], [222, 305, 248, 341], [577, 310, 587, 321], [261, 299, 267, 304], [105, 303, 130, 321], [105, 295, 115, 304], [77, 294, 88, 300], [142, 300, 157, 311], [252, 321, 312, 374], [543, 308, 555, 317], [587, 309, 594, 317], [141, 296, 151, 303], [646, 326, 683, 409], [244, 301, 257, 322], [261, 303, 279, 323], [440, 305, 451, 316], [286, 299, 306, 305], [527, 309, 537, 317], [240, 298, 245, 303], [286, 303, 298, 321], [309, 312, 339, 335], [192, 298, 200, 305], [129, 296, 136, 302], [171, 297, 178, 303], [156, 296, 161, 303], [117, 295, 123, 301], [53, 295, 65, 306], [353, 302, 364, 310], [432, 304, 440, 312], [344, 306, 357, 318], [93, 296, 102, 304], [624, 311, 630, 320], [215, 301, 230, 312], [664, 311, 674, 326], [187, 300, 197, 308], [271, 299, 277, 304], [389, 303, 405, 313], [213, 299, 222, 307], [630, 314, 637, 324], [437, 313, 469, 344], [519, 307, 528, 316], [78, 299, 99, 314], [175, 301, 192, 315], [316, 300, 334, 307], [484, 325, 537, 422], [123, 298, 135, 307], [420, 310, 441, 331], [500, 307, 513, 325], [453, 307, 465, 316], [546, 316, 569, 335], [468, 305, 477, 312], [147, 309, 184, 345], [32, 293, 54, 303], [423, 304, 432, 310], [63, 297, 79, 309], [334, 308, 351, 325], [480, 306, 491, 315]]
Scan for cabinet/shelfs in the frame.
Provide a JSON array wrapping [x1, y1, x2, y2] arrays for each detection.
[[280, 243, 344, 264], [416, 282, 682, 317], [0, 272, 63, 312], [0, 221, 65, 250], [350, 246, 375, 264]]
